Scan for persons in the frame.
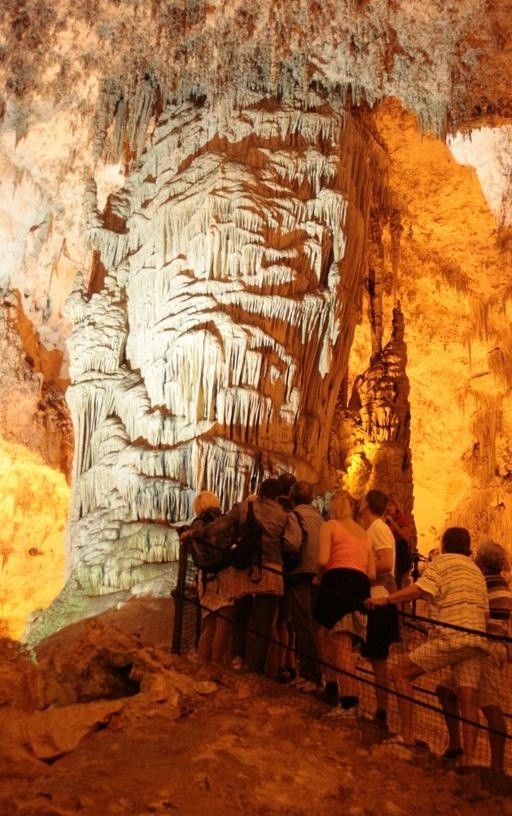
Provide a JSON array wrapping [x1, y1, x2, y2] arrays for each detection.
[[435, 541, 512, 773], [363, 526, 491, 767], [178, 473, 413, 732]]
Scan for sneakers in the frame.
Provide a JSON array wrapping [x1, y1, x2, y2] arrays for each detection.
[[234, 656, 338, 705], [440, 747, 464, 761], [382, 734, 416, 746], [322, 704, 357, 721]]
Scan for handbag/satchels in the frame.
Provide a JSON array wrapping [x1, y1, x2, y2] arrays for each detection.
[[279, 508, 309, 568]]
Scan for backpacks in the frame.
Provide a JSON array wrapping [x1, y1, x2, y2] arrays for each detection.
[[188, 512, 231, 573], [230, 501, 262, 570]]
